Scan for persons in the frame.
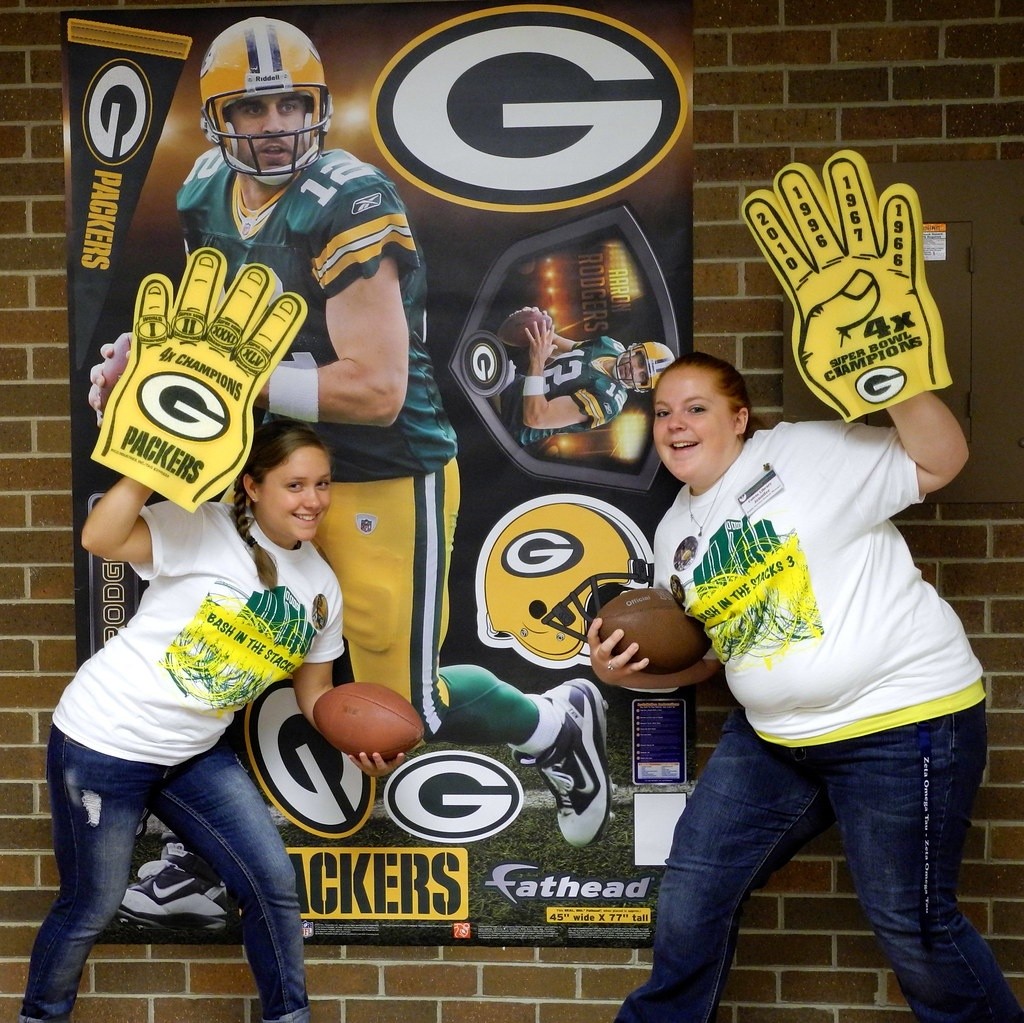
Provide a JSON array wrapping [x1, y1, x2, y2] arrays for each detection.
[[19, 419, 405, 1023], [491, 306, 675, 448], [588, 353, 1024, 1023], [89, 17, 618, 848]]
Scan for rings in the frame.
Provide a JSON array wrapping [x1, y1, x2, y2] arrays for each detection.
[[607, 661, 614, 672]]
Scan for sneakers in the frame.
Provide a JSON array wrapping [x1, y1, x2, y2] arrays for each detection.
[[105, 831, 227, 938], [512, 677, 618, 848]]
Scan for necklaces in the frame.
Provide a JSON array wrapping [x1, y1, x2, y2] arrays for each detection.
[[689, 477, 724, 537]]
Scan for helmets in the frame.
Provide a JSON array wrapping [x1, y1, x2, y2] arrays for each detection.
[[199, 17, 333, 185], [612, 342, 675, 394]]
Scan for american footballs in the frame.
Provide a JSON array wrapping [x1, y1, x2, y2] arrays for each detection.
[[599, 588, 713, 673], [100, 333, 133, 419], [497, 310, 552, 346], [312, 683, 424, 760]]
